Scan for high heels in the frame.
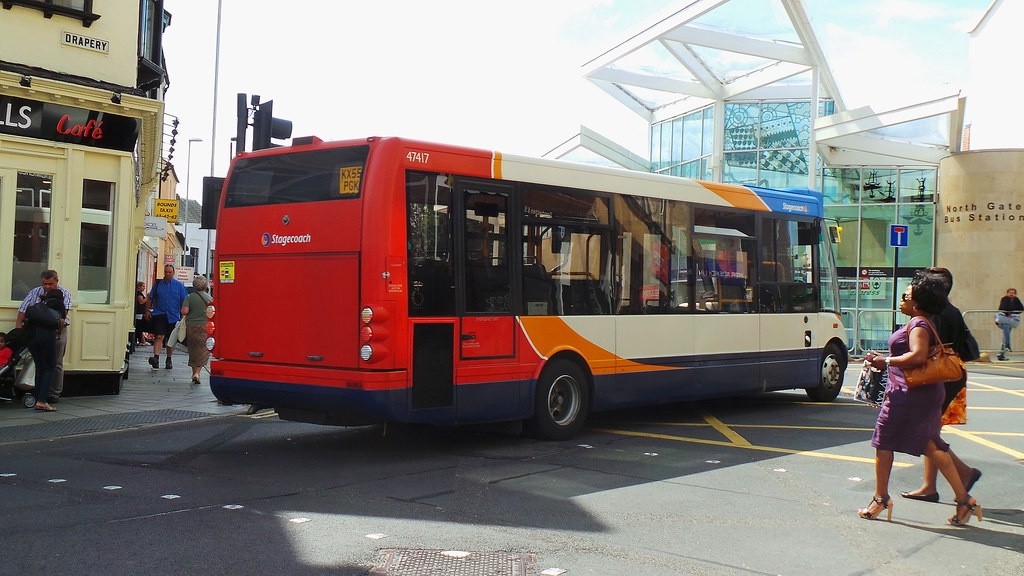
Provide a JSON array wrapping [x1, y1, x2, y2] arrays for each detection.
[[947, 496, 982, 526], [858, 495, 893, 521]]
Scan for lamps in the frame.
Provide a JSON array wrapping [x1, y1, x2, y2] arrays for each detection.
[[18, 74, 32, 87], [110, 92, 121, 104], [155, 112, 179, 182], [864, 172, 934, 235]]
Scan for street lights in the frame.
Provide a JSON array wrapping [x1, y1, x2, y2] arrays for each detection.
[[182, 138, 204, 267]]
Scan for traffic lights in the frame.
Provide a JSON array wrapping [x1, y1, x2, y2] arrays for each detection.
[[252, 100, 293, 151]]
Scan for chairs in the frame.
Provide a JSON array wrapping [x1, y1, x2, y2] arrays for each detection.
[[408, 251, 621, 316], [747, 280, 783, 311]]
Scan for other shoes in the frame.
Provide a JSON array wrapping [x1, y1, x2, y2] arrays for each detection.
[[35, 402, 56, 411], [166, 362, 172, 369], [192, 373, 201, 384], [149, 357, 159, 369], [1005, 345, 1013, 352], [139, 342, 151, 346], [997, 354, 1009, 360], [953, 468, 982, 503], [900, 491, 939, 503]]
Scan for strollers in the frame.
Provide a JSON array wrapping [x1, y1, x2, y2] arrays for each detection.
[[0, 327, 38, 408]]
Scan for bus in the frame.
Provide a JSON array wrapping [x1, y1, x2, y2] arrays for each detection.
[[205, 136, 851, 442]]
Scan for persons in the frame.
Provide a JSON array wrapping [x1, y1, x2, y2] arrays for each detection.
[[858, 267, 982, 525], [0, 332, 12, 369], [996, 288, 1024, 361], [17, 270, 71, 410], [137, 264, 213, 383]]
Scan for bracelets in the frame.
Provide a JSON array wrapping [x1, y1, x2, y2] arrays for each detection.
[[884, 358, 891, 366]]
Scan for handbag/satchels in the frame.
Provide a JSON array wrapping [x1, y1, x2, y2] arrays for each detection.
[[854, 360, 889, 408], [14, 359, 36, 391], [141, 313, 152, 332], [178, 316, 186, 342], [942, 357, 967, 425], [166, 315, 185, 348], [902, 316, 963, 389], [996, 313, 1020, 328]]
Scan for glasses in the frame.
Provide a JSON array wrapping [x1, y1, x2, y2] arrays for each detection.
[[901, 294, 912, 302]]
